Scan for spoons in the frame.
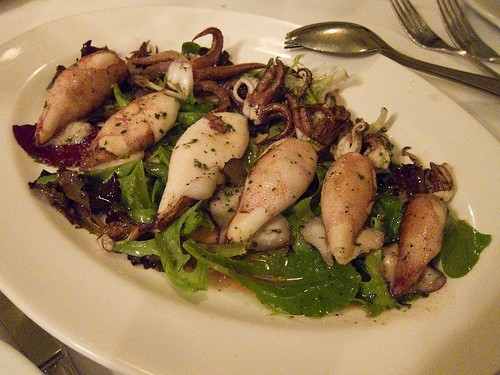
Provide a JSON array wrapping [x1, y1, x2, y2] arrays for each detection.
[[287, 22, 500, 96]]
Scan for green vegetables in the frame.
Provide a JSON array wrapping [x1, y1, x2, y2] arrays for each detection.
[[24, 39, 492, 318]]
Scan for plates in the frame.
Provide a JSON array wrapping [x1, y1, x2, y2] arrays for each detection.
[[0, 6, 500, 375]]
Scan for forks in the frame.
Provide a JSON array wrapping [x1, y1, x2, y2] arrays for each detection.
[[437, 0, 500, 66], [388, 0, 500, 80]]
